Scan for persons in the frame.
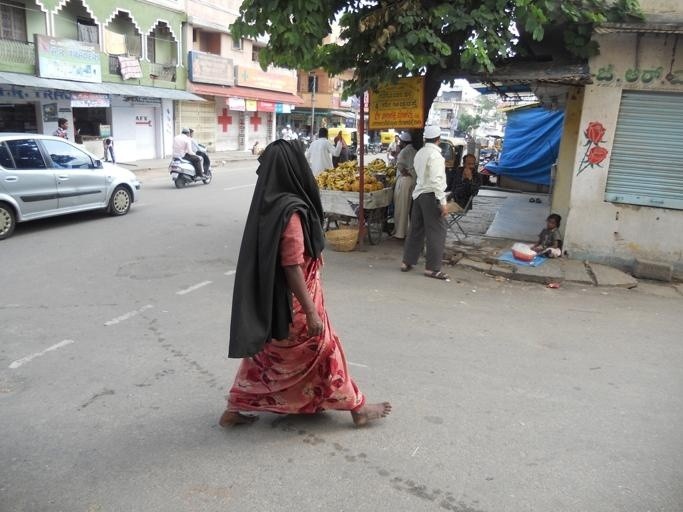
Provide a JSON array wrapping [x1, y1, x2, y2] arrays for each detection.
[[281, 124, 483, 280], [172, 127, 208, 181], [73, 117, 80, 142], [188, 128, 210, 175], [102, 137, 115, 164], [52, 118, 67, 141], [219, 138, 392, 428], [531, 214, 562, 259]]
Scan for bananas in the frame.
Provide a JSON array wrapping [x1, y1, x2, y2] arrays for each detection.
[[315, 158, 397, 191]]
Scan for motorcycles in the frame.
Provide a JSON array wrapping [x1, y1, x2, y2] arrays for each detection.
[[422, 135, 465, 172], [168, 141, 212, 189], [295, 127, 399, 166]]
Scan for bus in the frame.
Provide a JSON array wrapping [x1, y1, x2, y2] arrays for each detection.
[[482, 109, 500, 131]]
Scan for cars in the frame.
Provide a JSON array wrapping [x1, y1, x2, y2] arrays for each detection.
[[0, 132, 142, 239]]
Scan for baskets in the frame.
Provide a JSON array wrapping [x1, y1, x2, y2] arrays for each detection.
[[324, 227, 362, 251]]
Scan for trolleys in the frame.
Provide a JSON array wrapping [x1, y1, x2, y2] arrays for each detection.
[[316, 186, 396, 247]]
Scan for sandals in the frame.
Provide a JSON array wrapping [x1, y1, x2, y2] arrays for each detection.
[[400, 261, 414, 273], [424, 268, 450, 280]]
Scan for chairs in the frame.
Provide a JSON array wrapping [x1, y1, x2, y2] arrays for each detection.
[[437, 194, 478, 241]]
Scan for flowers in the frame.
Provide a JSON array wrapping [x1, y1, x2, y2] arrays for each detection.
[[577, 147, 611, 175], [575, 118, 607, 175]]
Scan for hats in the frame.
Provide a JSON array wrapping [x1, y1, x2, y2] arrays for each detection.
[[398, 132, 414, 142], [424, 125, 443, 139], [182, 127, 195, 134]]
